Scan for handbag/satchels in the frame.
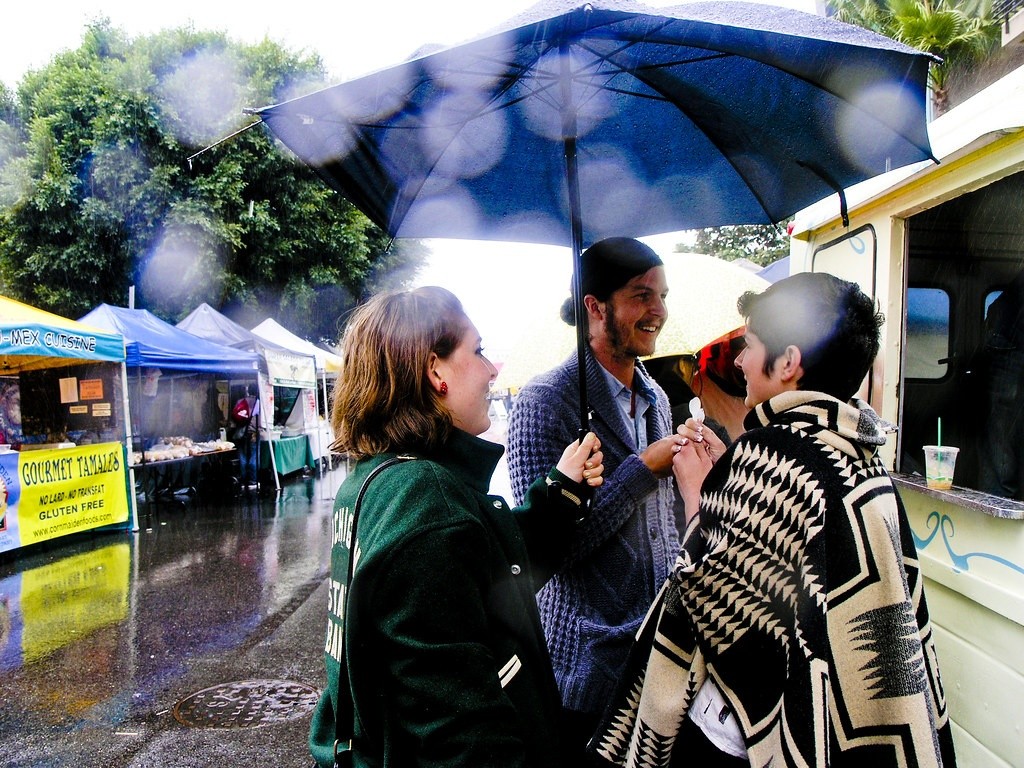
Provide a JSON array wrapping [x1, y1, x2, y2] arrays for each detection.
[[233, 399, 250, 421]]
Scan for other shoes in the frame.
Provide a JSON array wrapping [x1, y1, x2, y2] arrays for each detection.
[[249, 482, 260, 490]]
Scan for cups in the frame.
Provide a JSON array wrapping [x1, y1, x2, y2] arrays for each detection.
[[922, 445, 960, 490]]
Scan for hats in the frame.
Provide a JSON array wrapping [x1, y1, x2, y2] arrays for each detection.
[[206, 388, 221, 395]]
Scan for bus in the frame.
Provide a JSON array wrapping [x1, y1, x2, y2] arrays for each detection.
[[785, 65, 1024, 765]]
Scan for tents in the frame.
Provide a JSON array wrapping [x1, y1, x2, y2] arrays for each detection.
[[0, 293, 140, 554], [251, 318, 350, 470], [175, 302, 322, 474], [76, 302, 282, 513]]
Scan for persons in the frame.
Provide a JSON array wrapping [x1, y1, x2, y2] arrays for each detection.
[[201, 388, 225, 441], [507, 238, 680, 768], [594, 272, 958, 768], [233, 383, 260, 488], [307, 283, 603, 768]]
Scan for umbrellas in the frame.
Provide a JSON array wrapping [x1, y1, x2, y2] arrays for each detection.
[[240, 1, 945, 526]]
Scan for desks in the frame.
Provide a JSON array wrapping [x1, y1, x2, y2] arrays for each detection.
[[260, 434, 317, 498], [131, 447, 242, 528]]
